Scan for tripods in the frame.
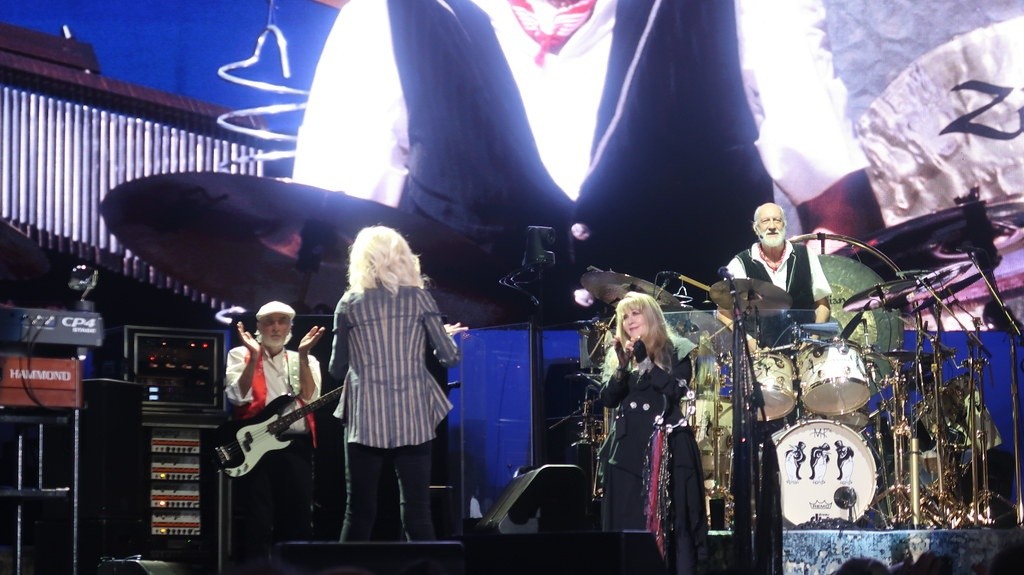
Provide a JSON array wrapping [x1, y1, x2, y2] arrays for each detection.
[[862, 276, 1015, 529]]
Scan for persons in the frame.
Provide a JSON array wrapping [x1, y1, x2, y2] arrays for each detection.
[[292, 0, 865, 329], [223, 296, 327, 563], [599, 291, 707, 575], [717, 202, 831, 356], [327, 225, 470, 544]]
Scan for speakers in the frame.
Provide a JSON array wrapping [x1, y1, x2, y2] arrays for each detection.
[[460, 322, 539, 518], [33, 379, 204, 575], [478, 463, 593, 534]]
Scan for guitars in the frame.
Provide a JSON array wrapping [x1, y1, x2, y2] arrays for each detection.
[[210, 383, 345, 482]]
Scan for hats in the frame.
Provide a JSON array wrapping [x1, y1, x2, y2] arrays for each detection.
[[255, 301, 297, 321]]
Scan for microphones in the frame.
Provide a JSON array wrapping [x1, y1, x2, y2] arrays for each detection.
[[663, 271, 711, 291], [896, 270, 932, 276], [956, 245, 984, 252], [718, 266, 732, 279], [839, 301, 871, 339], [755, 227, 764, 239]]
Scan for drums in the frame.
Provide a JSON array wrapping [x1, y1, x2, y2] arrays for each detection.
[[794, 336, 872, 416], [679, 394, 733, 498], [747, 351, 796, 422], [770, 418, 879, 530]]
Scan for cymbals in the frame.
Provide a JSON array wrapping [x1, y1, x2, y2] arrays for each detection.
[[96, 168, 527, 325], [579, 270, 682, 313], [826, 191, 1024, 282], [707, 276, 794, 318], [911, 260, 974, 295], [909, 265, 996, 315], [840, 273, 921, 314], [882, 350, 949, 363]]
